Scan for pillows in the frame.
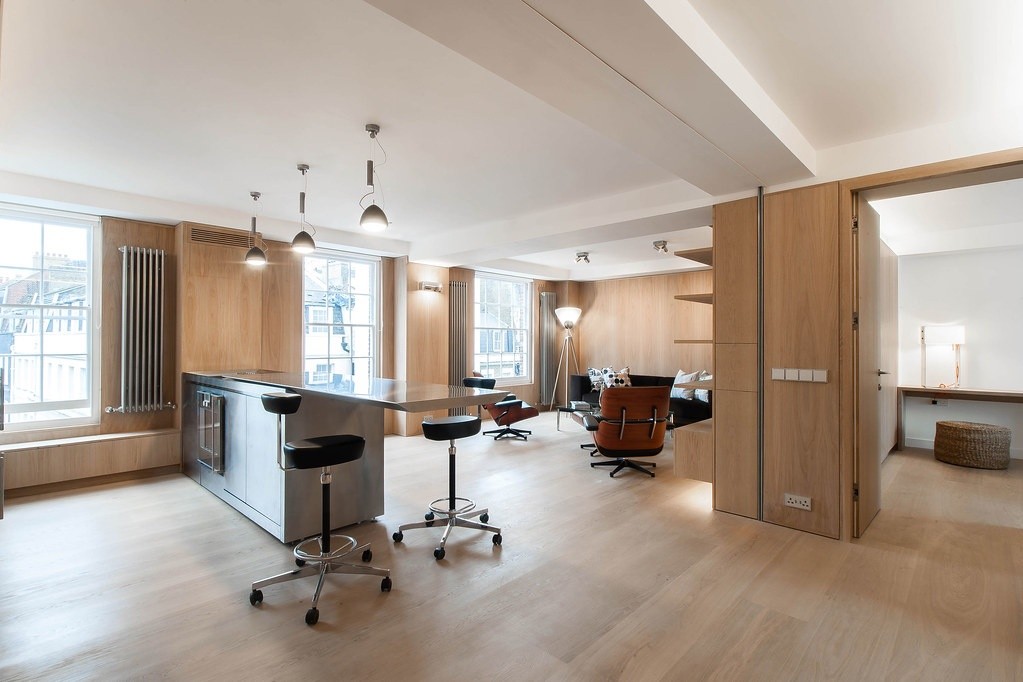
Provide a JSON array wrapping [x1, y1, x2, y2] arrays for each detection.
[[671, 369, 701, 400], [694, 369, 717, 404], [604, 367, 633, 389], [587, 365, 617, 391]]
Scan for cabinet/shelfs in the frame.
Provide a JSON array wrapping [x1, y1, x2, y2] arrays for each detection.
[[674, 245, 713, 390]]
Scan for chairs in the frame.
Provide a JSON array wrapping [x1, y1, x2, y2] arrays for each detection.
[[574, 386, 673, 479], [474, 371, 539, 440]]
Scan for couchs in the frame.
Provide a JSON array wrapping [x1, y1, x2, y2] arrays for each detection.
[[570, 373, 715, 429]]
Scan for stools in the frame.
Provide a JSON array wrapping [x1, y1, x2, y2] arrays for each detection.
[[391, 378, 500, 558], [250, 392, 392, 628], [932, 419, 1012, 469]]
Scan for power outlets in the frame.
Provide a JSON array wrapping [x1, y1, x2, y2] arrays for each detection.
[[783, 492, 812, 509], [932, 399, 949, 407]]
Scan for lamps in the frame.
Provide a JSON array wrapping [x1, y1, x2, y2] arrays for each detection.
[[246, 191, 266, 264], [291, 164, 317, 253], [653, 241, 667, 253], [550, 306, 588, 414], [420, 280, 442, 293], [576, 251, 590, 263], [358, 124, 389, 229]]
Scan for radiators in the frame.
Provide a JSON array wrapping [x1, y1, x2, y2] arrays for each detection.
[[116, 245, 165, 413]]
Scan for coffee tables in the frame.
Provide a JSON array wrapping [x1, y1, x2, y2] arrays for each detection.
[[557, 403, 600, 430]]
